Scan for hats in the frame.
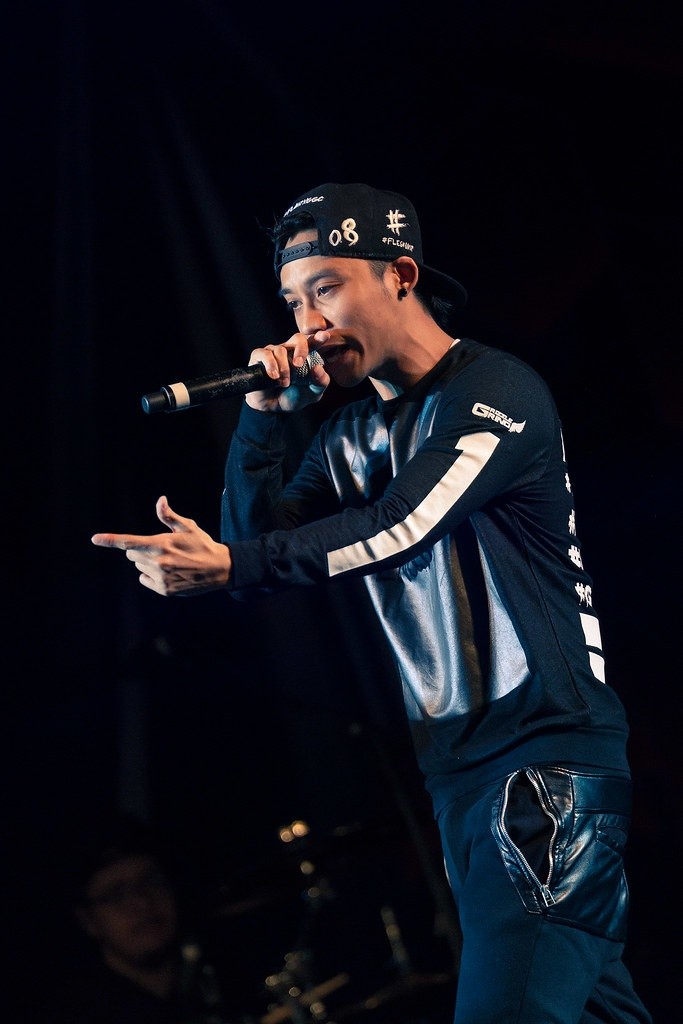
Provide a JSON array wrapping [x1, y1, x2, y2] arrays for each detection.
[[274, 184, 468, 305]]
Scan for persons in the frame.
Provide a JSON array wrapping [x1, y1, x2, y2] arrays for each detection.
[[90, 182, 650, 1023], [67, 816, 238, 1021]]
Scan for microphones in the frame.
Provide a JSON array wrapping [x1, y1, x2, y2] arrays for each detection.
[[143, 349, 325, 414]]
